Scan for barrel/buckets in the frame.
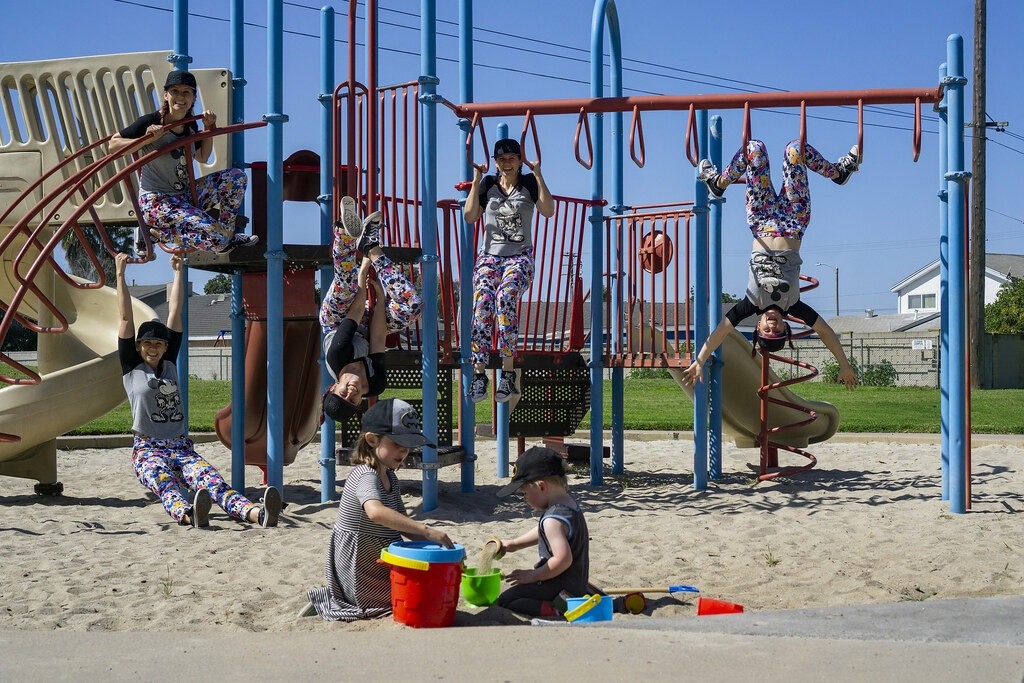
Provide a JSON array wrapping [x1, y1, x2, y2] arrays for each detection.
[[697, 597, 744, 617], [564, 594, 613, 622], [460, 567, 504, 605], [379, 541, 466, 627]]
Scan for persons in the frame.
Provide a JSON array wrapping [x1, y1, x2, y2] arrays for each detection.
[[113, 252, 281, 531], [494, 445, 607, 620], [320, 196, 424, 424], [297, 396, 455, 623], [108, 69, 259, 255], [681, 140, 860, 392], [464, 139, 554, 403]]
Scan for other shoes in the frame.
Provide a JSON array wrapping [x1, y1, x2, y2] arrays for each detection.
[[549, 589, 576, 615], [579, 583, 608, 597]]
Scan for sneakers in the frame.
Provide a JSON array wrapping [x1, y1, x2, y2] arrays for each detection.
[[698, 159, 727, 198], [133, 226, 160, 261], [469, 370, 489, 403], [187, 488, 212, 530], [297, 601, 318, 618], [494, 370, 517, 403], [830, 144, 860, 186], [340, 196, 363, 239], [259, 487, 281, 529], [218, 233, 259, 256], [355, 210, 382, 257]]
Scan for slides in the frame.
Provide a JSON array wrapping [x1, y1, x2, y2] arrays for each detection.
[[633, 320, 838, 450], [0, 225, 161, 484], [214, 315, 327, 465]]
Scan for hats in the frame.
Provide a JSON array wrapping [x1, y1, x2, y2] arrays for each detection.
[[490, 138, 521, 157], [324, 392, 360, 424], [163, 69, 198, 91], [137, 321, 169, 343], [757, 334, 787, 352], [496, 446, 565, 497], [360, 397, 437, 449]]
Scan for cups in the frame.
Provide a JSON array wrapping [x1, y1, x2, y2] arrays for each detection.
[[485, 539, 506, 561]]
